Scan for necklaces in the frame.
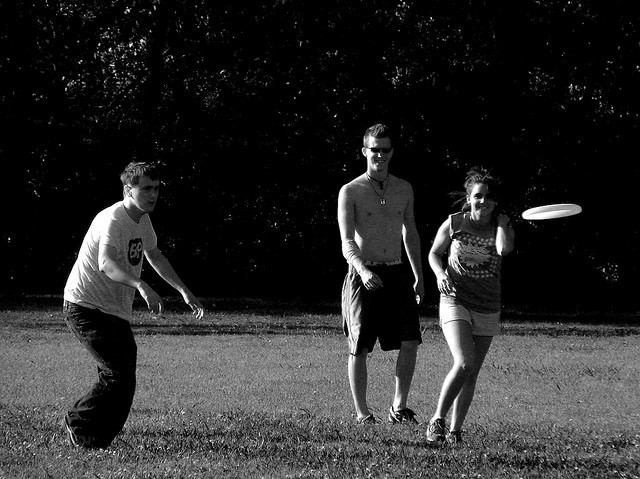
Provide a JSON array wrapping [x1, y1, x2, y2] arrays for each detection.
[[366, 172, 389, 205]]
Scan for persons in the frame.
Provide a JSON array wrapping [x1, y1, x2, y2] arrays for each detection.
[[427, 167, 514, 443], [338, 124, 424, 425], [64, 161, 204, 448]]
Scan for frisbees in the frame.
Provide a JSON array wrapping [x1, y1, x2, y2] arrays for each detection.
[[522, 203, 582, 220]]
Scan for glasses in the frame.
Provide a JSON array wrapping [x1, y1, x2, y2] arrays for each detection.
[[368, 143, 394, 154]]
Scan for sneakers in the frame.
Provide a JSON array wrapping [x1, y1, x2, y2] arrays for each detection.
[[357, 414, 375, 424], [388, 406, 420, 425], [426, 417, 448, 448], [63, 414, 84, 450], [447, 430, 464, 445]]
